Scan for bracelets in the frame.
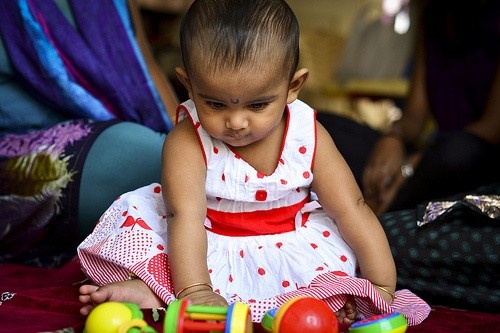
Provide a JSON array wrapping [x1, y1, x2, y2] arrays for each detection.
[[374, 284, 395, 301], [174, 283, 212, 300]]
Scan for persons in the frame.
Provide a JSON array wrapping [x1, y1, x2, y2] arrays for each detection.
[[77, 0, 431, 326], [312, 0, 500, 221]]
